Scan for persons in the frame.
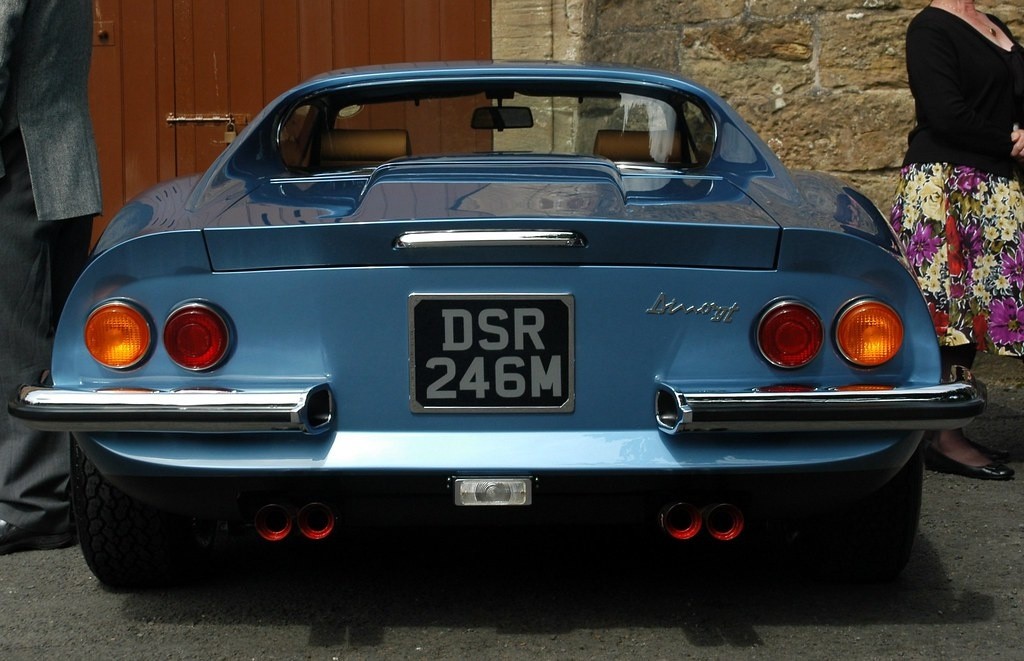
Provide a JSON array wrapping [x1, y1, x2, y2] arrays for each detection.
[[890, 0, 1024, 480], [0, 0, 104, 553]]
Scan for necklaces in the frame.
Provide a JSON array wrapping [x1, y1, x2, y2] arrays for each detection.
[[936, 1, 996, 36]]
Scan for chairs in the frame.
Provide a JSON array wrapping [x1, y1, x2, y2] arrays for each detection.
[[317, 129, 412, 170], [593, 130, 681, 162]]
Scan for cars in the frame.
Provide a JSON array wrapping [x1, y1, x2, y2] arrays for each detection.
[[7, 59, 992, 600]]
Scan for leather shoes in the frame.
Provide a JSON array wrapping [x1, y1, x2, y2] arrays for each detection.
[[925, 444, 1015, 478]]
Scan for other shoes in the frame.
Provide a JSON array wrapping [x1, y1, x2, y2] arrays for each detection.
[[0, 518, 74, 554]]
